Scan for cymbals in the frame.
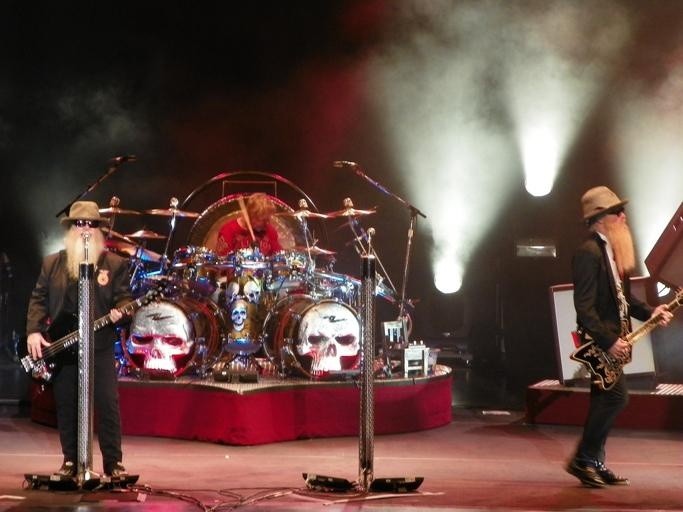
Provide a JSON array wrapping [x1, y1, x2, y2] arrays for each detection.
[[145, 208, 202, 220], [292, 244, 337, 256], [104, 238, 171, 265], [125, 229, 171, 243], [325, 208, 377, 219], [99, 209, 141, 216], [274, 210, 329, 219]]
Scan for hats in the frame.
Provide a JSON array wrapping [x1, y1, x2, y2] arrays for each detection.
[[61, 201, 110, 228], [580, 186, 628, 220]]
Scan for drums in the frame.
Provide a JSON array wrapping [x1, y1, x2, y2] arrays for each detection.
[[173, 246, 352, 300], [119, 288, 227, 379], [221, 297, 264, 354], [258, 299, 365, 380]]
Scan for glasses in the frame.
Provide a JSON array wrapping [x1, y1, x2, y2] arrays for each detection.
[[609, 208, 624, 217], [71, 220, 100, 227]]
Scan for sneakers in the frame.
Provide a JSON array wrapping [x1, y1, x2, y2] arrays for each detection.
[[597, 462, 630, 484], [58, 459, 76, 476], [566, 457, 603, 487], [112, 461, 128, 477]]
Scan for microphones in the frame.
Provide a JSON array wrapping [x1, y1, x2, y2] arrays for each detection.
[[333, 160, 359, 169], [367, 227, 376, 252], [83, 230, 91, 262], [114, 154, 135, 163]]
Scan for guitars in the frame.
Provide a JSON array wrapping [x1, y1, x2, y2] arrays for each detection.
[[16, 287, 168, 386], [571, 285, 683, 394]]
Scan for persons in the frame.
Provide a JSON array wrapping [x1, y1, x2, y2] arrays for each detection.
[[26, 200, 134, 476], [562, 184, 674, 488], [214, 193, 283, 257]]
